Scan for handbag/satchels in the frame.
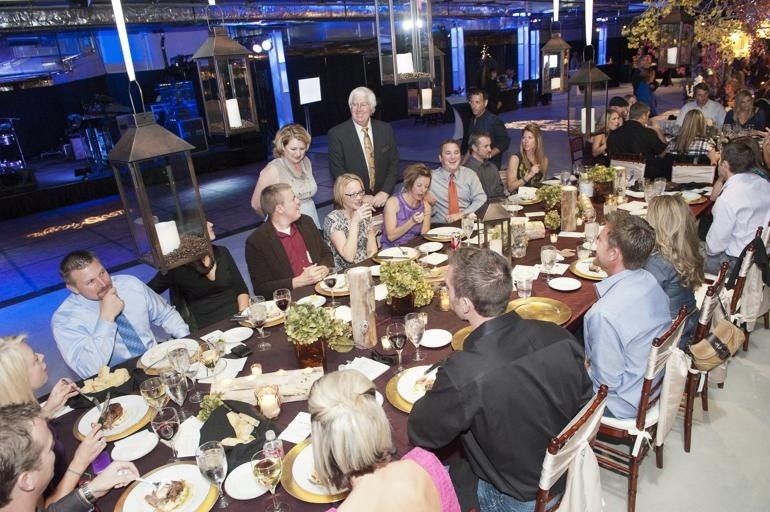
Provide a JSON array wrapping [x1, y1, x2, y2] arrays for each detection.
[[688, 290, 746, 372]]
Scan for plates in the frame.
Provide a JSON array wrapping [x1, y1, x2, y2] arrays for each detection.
[[575, 259, 608, 278], [222, 327, 254, 342], [418, 241, 444, 252], [140, 338, 198, 370], [372, 246, 420, 264], [548, 276, 582, 292], [241, 300, 284, 322], [369, 265, 382, 276], [72, 393, 155, 442], [378, 246, 416, 261], [224, 461, 267, 500], [451, 324, 471, 352], [110, 431, 159, 462], [314, 280, 350, 296], [296, 294, 326, 308], [114, 461, 221, 512], [292, 442, 349, 495], [420, 328, 452, 348], [509, 194, 542, 205], [397, 365, 439, 403], [569, 258, 609, 281], [279, 437, 352, 504], [682, 193, 702, 201], [422, 235, 457, 242], [506, 296, 572, 325], [236, 302, 297, 329], [385, 365, 435, 414], [680, 192, 707, 205], [321, 273, 350, 292], [428, 226, 463, 239], [135, 339, 208, 375], [77, 394, 150, 438]]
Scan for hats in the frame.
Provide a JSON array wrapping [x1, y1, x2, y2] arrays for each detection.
[[609, 97, 629, 107]]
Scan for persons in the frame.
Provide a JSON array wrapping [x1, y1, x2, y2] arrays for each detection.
[[407, 248, 594, 511], [49, 248, 192, 380], [0, 335, 141, 512], [324, 173, 381, 270], [695, 133, 770, 327], [642, 192, 706, 349], [306, 370, 464, 512], [326, 85, 403, 215], [0, 399, 141, 512], [460, 87, 512, 170], [571, 211, 673, 419], [244, 183, 336, 301], [382, 122, 548, 250], [505, 69, 518, 87], [484, 67, 502, 116], [248, 122, 324, 241], [582, 52, 769, 182], [145, 208, 251, 331]]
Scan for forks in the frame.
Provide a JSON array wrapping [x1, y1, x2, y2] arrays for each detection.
[[62, 378, 101, 412], [117, 466, 171, 489]]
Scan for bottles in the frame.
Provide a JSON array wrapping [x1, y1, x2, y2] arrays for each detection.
[[263, 429, 285, 461]]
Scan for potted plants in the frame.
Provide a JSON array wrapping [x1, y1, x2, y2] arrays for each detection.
[[378, 259, 435, 319], [282, 301, 353, 372]]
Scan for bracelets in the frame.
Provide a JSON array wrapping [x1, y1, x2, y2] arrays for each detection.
[[78, 480, 98, 505], [522, 177, 528, 183]]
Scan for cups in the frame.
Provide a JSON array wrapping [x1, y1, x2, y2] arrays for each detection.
[[254, 385, 282, 420], [505, 196, 519, 218], [561, 170, 570, 186], [644, 185, 654, 206], [654, 175, 666, 197], [516, 270, 534, 298], [510, 219, 528, 259]]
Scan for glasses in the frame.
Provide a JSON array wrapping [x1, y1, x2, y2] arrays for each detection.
[[371, 349, 398, 365], [345, 190, 365, 198]]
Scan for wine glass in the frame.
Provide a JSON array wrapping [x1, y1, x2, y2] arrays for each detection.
[[461, 212, 475, 249], [140, 376, 170, 414], [248, 295, 271, 339], [177, 352, 208, 403], [404, 312, 425, 362], [248, 305, 271, 352], [150, 407, 181, 465], [272, 288, 292, 330], [387, 322, 408, 373], [251, 450, 290, 512], [167, 345, 194, 391], [161, 373, 188, 422], [322, 269, 341, 309], [195, 440, 231, 509], [540, 245, 557, 283], [199, 344, 222, 384], [585, 222, 599, 256]]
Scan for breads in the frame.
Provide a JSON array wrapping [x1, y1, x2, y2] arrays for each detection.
[[145, 478, 186, 512], [101, 402, 123, 429]]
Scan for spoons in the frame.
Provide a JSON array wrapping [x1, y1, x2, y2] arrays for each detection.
[[391, 242, 409, 255]]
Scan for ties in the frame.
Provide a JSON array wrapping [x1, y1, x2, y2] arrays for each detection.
[[362, 127, 376, 193], [448, 175, 461, 226], [115, 313, 147, 357]]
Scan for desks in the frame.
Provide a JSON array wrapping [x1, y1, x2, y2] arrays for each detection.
[[34, 165, 716, 512]]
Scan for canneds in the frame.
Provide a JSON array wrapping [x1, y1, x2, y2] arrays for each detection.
[[451, 232, 460, 249]]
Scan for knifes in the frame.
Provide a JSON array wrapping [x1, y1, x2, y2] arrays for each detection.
[[375, 255, 409, 261], [97, 391, 111, 428], [424, 354, 448, 376]]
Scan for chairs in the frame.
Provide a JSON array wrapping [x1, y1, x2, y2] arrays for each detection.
[[568, 96, 770, 186], [371, 209, 387, 250], [169, 284, 199, 335], [536, 226, 770, 509]]
